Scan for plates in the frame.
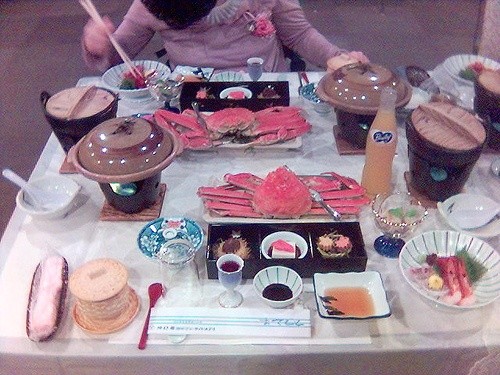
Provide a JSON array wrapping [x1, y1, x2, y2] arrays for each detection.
[[313, 270, 392, 320], [168, 64, 215, 82], [72, 286, 140, 335], [136, 216, 204, 259], [180, 82, 500, 279]]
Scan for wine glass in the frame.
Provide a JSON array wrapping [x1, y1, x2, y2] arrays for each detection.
[[372, 190, 427, 259], [216, 253, 243, 308]]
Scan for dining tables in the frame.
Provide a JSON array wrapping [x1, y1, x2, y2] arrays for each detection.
[[0, 71, 500, 375]]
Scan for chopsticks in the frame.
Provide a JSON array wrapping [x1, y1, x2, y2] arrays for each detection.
[[79, 0, 144, 79]]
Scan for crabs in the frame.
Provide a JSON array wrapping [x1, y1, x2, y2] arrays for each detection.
[[152, 106, 314, 148], [196, 163, 371, 220]]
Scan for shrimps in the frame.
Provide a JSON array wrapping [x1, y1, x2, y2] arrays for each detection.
[[457, 262, 474, 306], [436, 258, 462, 305]]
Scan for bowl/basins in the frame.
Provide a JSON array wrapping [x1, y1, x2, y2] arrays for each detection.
[[15, 175, 82, 221], [398, 192, 500, 308], [396, 86, 432, 118], [443, 54, 500, 85], [101, 60, 171, 98], [252, 265, 303, 308], [213, 71, 244, 81]]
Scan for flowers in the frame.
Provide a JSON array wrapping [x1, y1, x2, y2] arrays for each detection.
[[244, 12, 277, 38]]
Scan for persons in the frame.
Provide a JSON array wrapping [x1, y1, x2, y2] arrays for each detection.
[[81, 0, 368, 76]]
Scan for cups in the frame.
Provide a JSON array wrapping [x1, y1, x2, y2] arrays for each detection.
[[246, 57, 264, 82], [159, 240, 203, 307], [67, 259, 130, 321], [260, 231, 308, 259]]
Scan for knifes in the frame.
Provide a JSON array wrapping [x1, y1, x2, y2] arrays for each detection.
[[284, 164, 343, 222]]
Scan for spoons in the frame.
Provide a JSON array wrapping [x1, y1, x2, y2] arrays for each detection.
[[1, 168, 69, 209], [138, 283, 166, 350]]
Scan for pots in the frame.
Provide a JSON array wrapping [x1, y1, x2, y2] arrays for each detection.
[[316, 61, 500, 167], [40, 85, 183, 184]]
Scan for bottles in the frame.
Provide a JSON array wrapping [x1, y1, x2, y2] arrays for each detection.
[[360, 88, 398, 203]]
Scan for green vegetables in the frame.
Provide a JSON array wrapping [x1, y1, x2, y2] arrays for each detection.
[[458, 69, 476, 81], [455, 245, 486, 282], [389, 208, 417, 221], [119, 78, 135, 89]]
